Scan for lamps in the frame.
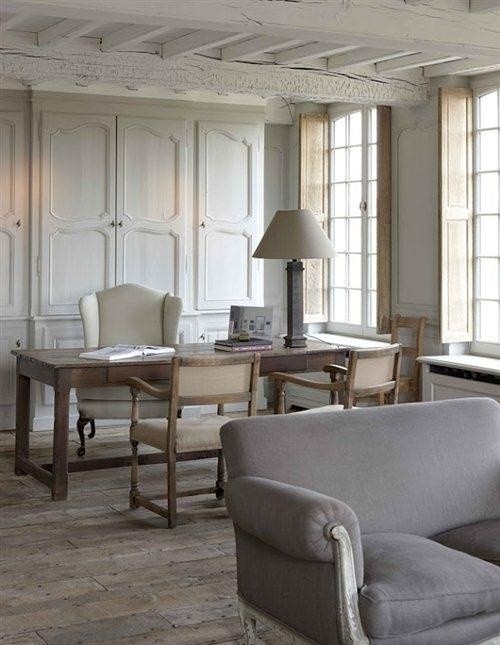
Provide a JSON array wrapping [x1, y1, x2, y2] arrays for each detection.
[[251, 209, 338, 347]]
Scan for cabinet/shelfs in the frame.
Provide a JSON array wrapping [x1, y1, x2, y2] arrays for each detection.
[[413, 354, 500, 402]]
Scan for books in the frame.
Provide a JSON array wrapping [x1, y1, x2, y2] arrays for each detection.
[[214, 335, 274, 352], [78, 342, 175, 361]]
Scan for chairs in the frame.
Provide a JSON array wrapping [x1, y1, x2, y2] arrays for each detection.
[[270, 342, 403, 414], [76, 282, 183, 454], [386, 313, 427, 403], [126, 351, 261, 530]]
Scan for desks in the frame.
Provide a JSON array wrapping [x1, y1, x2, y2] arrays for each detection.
[[10, 335, 391, 503]]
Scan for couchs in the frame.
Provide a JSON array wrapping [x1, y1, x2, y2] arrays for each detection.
[[219, 395, 500, 644]]
